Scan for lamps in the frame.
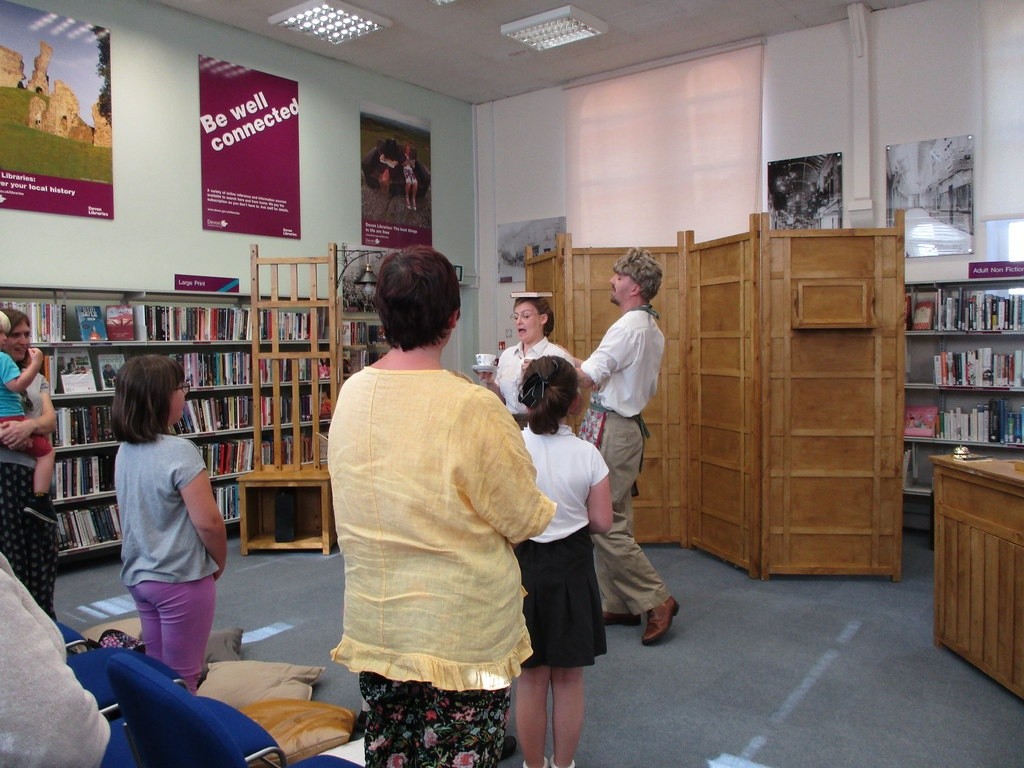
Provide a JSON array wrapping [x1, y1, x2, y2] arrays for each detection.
[[335, 246, 385, 295], [268, 0, 392, 45], [500, 4, 607, 52]]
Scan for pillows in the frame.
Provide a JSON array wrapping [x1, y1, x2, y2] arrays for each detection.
[[238, 699, 355, 768], [69, 618, 142, 653], [196, 661, 326, 708], [203, 628, 244, 676]]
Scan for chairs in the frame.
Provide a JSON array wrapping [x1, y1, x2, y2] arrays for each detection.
[[54, 621, 363, 768]]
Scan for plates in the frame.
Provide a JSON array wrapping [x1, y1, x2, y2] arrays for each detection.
[[470, 365, 499, 372]]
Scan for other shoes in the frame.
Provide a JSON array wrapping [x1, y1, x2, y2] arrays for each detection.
[[502, 735, 516, 761], [23, 493, 58, 526]]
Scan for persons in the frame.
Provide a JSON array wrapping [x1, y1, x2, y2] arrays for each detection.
[[569, 249, 679, 647], [0, 308, 60, 623], [325, 243, 550, 768], [111, 354, 227, 696], [1, 311, 59, 525], [473, 298, 609, 768]]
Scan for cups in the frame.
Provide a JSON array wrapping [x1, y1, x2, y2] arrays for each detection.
[[475, 354, 496, 366]]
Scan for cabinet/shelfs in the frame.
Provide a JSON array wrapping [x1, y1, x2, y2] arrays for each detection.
[[905, 277, 1024, 496], [0, 243, 391, 555]]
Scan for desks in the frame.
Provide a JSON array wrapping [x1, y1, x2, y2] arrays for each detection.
[[929, 454, 1024, 700]]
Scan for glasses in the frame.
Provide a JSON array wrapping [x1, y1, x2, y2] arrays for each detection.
[[21, 392, 33, 412], [509, 311, 549, 320], [174, 382, 190, 394]]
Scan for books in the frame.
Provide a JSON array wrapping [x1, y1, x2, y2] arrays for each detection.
[[903, 449, 913, 489], [934, 348, 1024, 387], [1, 299, 393, 552], [508, 291, 554, 299], [905, 287, 1024, 331]]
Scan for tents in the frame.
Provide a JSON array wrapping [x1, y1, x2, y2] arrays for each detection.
[[905, 397, 1024, 446]]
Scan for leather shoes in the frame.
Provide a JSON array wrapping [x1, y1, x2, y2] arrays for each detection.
[[641, 597, 679, 644], [604, 611, 641, 626]]
